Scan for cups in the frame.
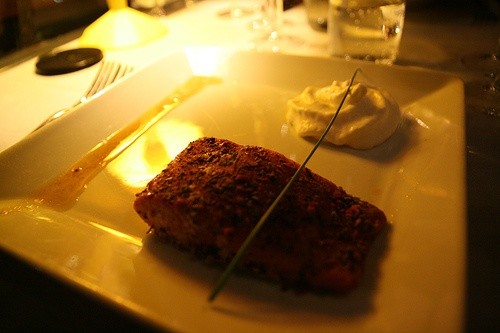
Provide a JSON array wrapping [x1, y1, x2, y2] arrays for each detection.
[[327, 0, 406, 65], [304, 0, 327, 33]]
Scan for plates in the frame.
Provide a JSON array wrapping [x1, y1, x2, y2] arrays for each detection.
[[0, 45, 466, 332]]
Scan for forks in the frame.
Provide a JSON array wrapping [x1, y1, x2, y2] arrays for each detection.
[[25, 60, 135, 138]]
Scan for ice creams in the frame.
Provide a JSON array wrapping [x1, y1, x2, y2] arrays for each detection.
[[286, 80, 402, 150]]
